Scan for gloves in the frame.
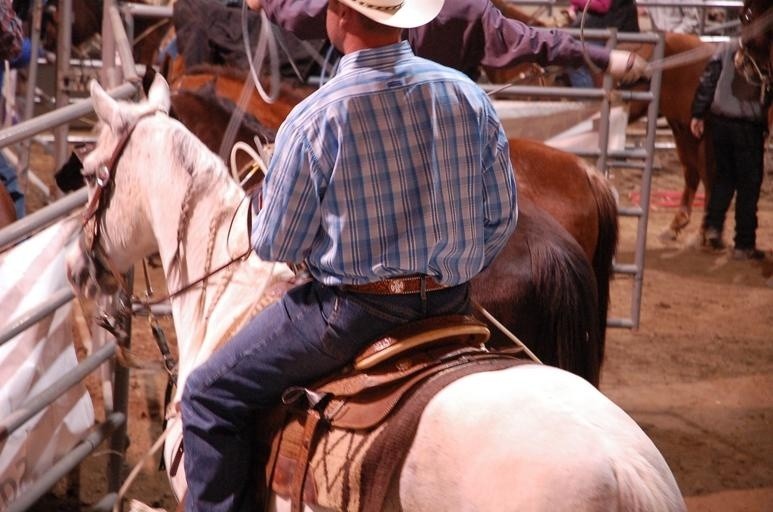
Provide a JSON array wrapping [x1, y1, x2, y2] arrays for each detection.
[[606, 46, 654, 85]]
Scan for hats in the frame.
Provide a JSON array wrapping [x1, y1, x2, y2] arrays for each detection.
[[331, 0, 449, 29]]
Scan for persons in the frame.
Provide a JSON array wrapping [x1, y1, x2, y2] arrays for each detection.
[[689, 33, 773, 259], [241, 0, 653, 84], [0, 0, 46, 248], [181, 0, 520, 512]]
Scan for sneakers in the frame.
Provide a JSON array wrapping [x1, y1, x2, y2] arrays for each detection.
[[701, 227, 729, 255], [733, 244, 767, 262]]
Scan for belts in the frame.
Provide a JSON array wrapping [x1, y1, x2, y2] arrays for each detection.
[[336, 273, 450, 297]]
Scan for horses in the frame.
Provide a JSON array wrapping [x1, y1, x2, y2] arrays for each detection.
[[729, 1, 773, 279], [66, 69, 686, 512], [161, 49, 620, 344], [141, 63, 602, 392], [481, 0, 713, 250]]
